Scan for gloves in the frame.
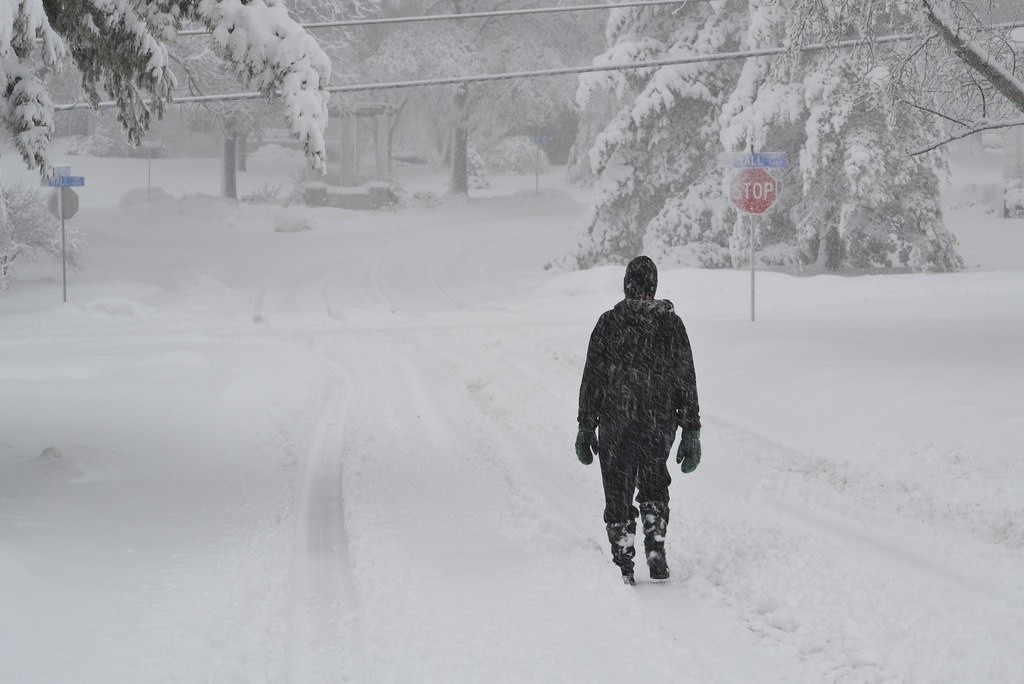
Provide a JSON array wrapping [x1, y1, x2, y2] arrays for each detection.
[[575, 425, 598, 465], [676, 430, 702, 474]]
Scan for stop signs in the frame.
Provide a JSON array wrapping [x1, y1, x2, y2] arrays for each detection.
[[731, 166, 777, 215]]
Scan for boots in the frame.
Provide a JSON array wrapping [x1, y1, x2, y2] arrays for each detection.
[[606, 520, 637, 587], [639, 500, 670, 582]]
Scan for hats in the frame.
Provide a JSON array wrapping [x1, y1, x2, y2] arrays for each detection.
[[624, 255, 658, 300]]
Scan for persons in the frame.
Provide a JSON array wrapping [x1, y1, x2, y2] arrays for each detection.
[[576, 256, 702, 585]]
